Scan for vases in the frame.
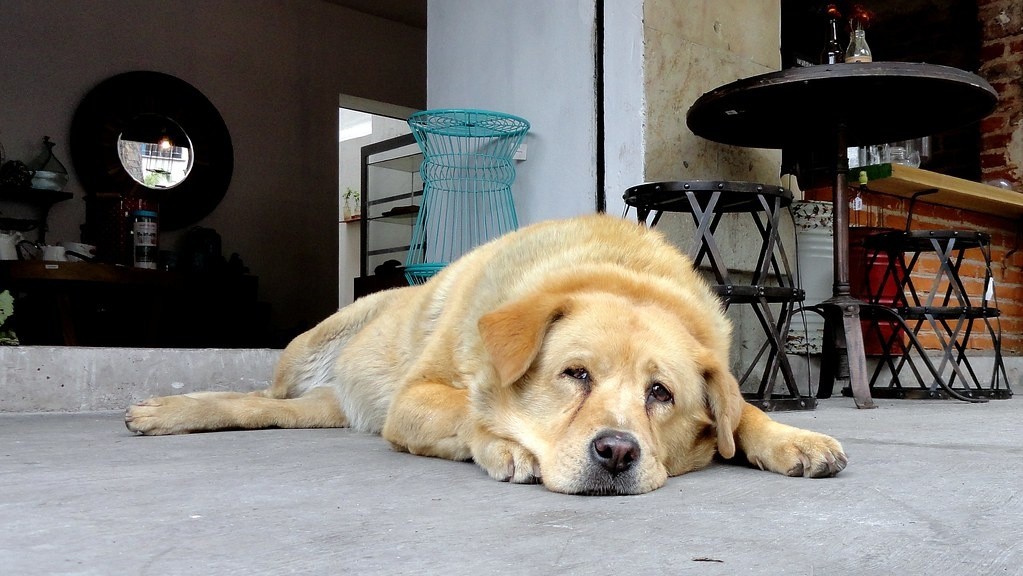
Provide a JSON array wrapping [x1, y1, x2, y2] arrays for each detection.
[[352, 203, 360, 219], [343, 203, 351, 219]]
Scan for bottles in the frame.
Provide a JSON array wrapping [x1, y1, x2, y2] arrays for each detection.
[[820, 18, 845, 64], [133, 210, 159, 270]]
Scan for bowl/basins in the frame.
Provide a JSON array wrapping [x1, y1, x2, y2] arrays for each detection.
[[61, 241, 97, 262]]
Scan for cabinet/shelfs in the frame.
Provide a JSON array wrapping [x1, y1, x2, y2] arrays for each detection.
[[361, 132, 428, 277]]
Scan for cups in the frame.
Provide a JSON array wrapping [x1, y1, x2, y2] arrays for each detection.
[[42, 245, 67, 262]]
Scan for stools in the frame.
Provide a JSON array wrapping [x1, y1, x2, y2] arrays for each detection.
[[841, 228, 1013, 400], [621, 180, 818, 413]]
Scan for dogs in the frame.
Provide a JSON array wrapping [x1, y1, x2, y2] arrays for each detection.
[[124, 216, 848, 496]]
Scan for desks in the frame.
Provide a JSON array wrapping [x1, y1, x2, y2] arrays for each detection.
[[0, 260, 170, 346], [685, 62, 1000, 410], [0, 183, 74, 258]]
